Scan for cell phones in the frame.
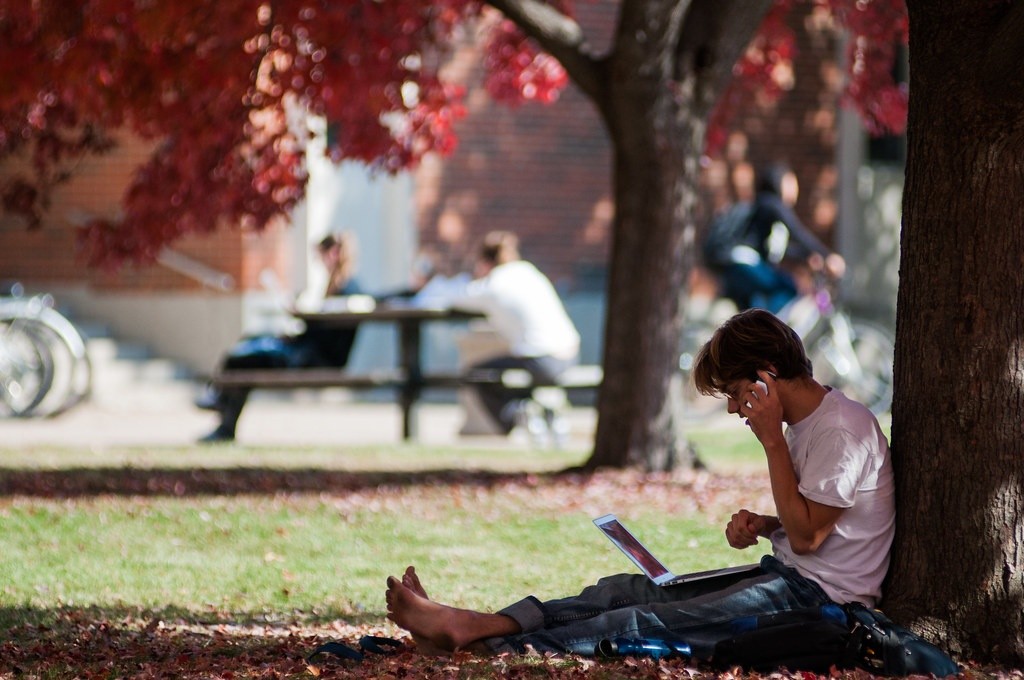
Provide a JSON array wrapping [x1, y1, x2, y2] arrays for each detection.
[[746, 371, 776, 409]]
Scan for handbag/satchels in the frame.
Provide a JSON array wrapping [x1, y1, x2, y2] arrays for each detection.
[[710, 609, 882, 676]]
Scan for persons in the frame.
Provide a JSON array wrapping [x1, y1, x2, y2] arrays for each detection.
[[718, 162, 845, 317], [192, 231, 367, 443], [441, 230, 580, 436], [385, 308, 895, 657]]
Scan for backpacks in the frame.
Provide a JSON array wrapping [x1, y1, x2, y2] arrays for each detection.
[[701, 200, 760, 267]]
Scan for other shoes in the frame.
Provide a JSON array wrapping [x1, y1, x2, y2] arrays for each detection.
[[199, 427, 236, 441], [193, 395, 229, 410]]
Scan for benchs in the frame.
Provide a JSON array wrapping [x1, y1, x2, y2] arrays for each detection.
[[207, 357, 605, 390]]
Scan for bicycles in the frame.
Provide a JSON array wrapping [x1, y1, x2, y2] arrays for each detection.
[[671, 254, 894, 425], [0, 285, 94, 423]]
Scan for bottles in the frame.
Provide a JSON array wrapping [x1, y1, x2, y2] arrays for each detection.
[[595, 637, 691, 664]]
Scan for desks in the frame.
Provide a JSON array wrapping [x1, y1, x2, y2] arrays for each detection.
[[283, 305, 488, 439]]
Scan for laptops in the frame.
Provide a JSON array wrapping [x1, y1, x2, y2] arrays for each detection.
[[592, 514, 760, 588]]
